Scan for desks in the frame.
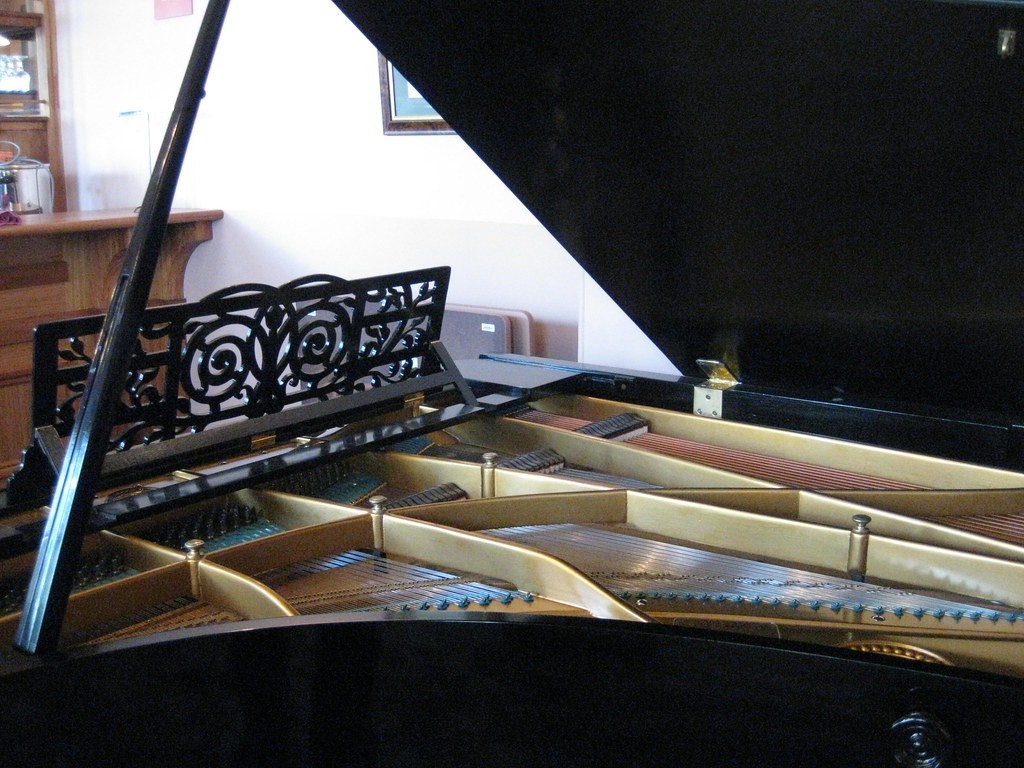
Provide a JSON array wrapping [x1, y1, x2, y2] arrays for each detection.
[[290, 295, 535, 405]]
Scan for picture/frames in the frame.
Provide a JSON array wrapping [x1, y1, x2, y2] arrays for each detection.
[[377, 50, 459, 136]]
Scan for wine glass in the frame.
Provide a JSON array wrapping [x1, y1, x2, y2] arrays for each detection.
[[0, 54, 31, 93]]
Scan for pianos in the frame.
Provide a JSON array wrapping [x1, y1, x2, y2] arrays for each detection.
[[0, 0, 1024, 768]]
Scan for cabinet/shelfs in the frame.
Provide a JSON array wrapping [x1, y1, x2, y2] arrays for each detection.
[[0, 0, 224, 489]]
